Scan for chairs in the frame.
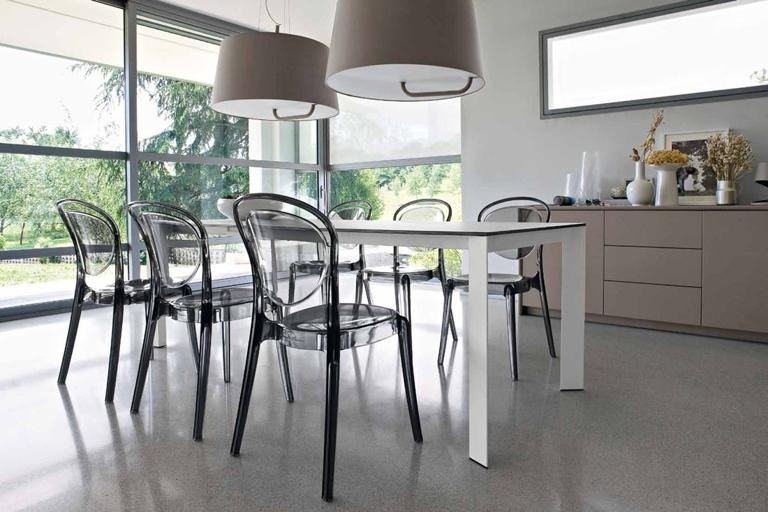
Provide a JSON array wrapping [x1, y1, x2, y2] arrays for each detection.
[[56, 200, 198, 402], [355, 199, 457, 342], [289, 201, 372, 305], [437, 196, 556, 381], [230, 193, 424, 503], [126, 204, 295, 442]]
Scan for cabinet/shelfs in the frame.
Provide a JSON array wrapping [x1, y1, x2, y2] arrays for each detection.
[[604, 206, 702, 335], [517, 206, 604, 322], [701, 206, 768, 340]]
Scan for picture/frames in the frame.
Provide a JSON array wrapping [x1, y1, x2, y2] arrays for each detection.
[[665, 130, 729, 204], [539, 1, 768, 119]]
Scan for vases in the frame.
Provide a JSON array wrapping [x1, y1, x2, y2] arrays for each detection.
[[648, 164, 684, 205], [716, 180, 736, 205], [627, 162, 654, 207]]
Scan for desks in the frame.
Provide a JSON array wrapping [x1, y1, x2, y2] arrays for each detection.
[[153, 220, 586, 468]]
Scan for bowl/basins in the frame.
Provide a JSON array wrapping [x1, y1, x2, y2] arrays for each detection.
[[216, 198, 282, 220]]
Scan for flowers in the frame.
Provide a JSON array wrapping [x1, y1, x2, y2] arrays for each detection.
[[645, 150, 688, 165], [628, 109, 665, 163], [703, 130, 751, 180]]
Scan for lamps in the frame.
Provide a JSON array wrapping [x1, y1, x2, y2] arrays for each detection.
[[210, 24, 339, 121], [325, 0, 484, 102]]
